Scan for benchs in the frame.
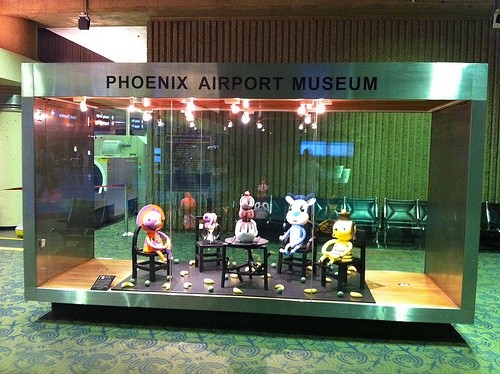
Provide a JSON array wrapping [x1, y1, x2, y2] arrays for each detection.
[[383, 198, 500, 250], [240, 194, 381, 248]]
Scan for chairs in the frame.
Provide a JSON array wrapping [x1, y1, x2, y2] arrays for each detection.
[[132, 225, 172, 282], [277, 220, 318, 278], [195, 216, 223, 273], [321, 229, 366, 292]]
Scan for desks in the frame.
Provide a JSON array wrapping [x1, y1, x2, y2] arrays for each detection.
[[221, 237, 271, 290]]
